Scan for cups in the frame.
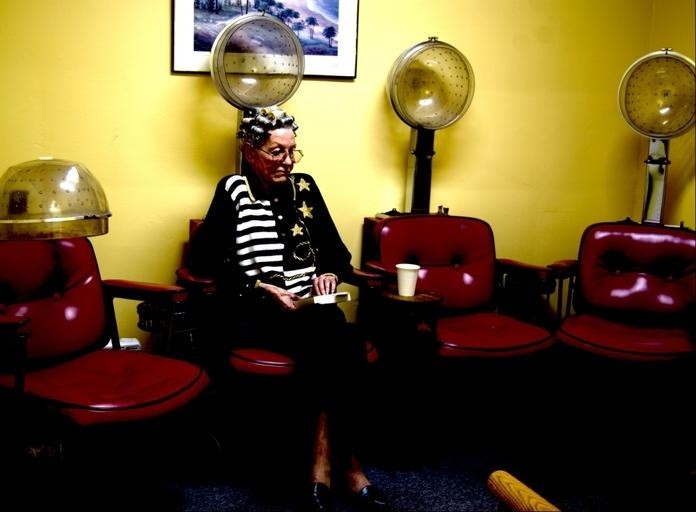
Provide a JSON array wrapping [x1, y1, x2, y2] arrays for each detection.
[[395, 263, 421, 297]]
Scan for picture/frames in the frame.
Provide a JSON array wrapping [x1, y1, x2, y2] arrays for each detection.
[[168, 1, 361, 82]]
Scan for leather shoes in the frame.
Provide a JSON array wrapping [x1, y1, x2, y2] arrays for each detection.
[[308, 481, 391, 511]]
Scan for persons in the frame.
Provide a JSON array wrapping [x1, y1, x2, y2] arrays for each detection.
[[183, 107, 392, 511]]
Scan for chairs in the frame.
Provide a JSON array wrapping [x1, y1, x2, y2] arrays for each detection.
[[365, 214, 559, 363], [175, 220, 378, 375], [3, 234, 210, 459], [548, 217, 694, 370]]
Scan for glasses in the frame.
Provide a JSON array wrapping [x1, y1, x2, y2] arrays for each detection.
[[254, 145, 303, 163]]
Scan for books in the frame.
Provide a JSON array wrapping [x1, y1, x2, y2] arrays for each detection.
[[293, 291, 353, 305]]
